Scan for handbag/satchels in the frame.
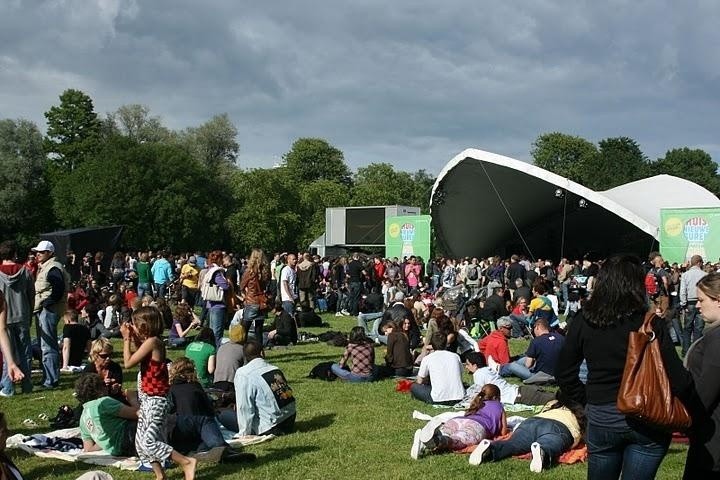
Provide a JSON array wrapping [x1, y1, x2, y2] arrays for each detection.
[[203, 283, 226, 302], [311, 361, 337, 381], [617, 331, 693, 430], [258, 291, 275, 311]]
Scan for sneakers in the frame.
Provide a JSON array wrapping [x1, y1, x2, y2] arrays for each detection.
[[468, 439, 490, 466], [530, 442, 543, 473], [223, 452, 256, 463], [411, 429, 426, 460], [193, 447, 229, 463], [420, 417, 443, 443]]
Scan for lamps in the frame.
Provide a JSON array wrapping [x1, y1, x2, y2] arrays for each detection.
[[552, 188, 589, 209]]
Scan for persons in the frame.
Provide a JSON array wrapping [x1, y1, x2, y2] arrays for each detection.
[[0, 241, 301, 480], [421, 259, 719, 478], [305, 247, 420, 384]]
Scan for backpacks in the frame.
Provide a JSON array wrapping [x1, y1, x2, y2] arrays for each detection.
[[645, 268, 662, 295], [466, 265, 480, 280]]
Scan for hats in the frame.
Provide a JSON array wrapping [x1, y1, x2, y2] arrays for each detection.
[[31, 241, 55, 252]]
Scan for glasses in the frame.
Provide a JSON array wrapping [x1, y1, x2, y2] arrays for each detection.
[[95, 352, 113, 358], [503, 326, 513, 330]]
